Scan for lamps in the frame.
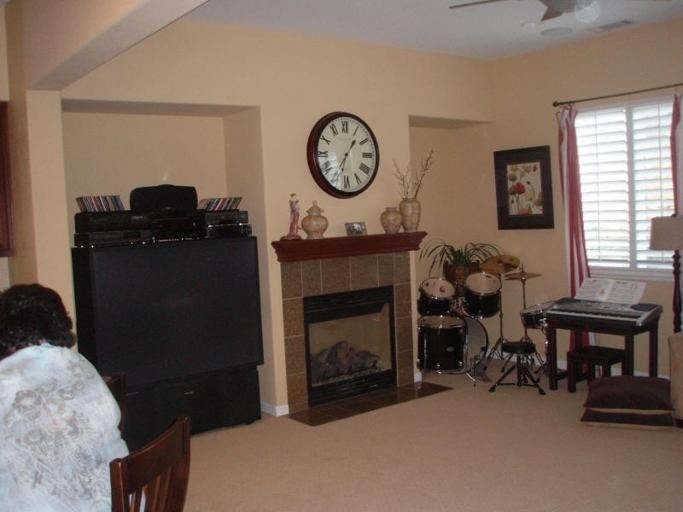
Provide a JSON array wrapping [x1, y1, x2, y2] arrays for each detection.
[[650, 213, 683, 335]]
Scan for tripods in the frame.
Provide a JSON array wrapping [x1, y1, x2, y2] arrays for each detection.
[[489, 356, 548, 397], [499, 287, 550, 373], [473, 276, 518, 371]]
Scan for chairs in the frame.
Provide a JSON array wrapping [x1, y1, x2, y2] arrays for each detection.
[[111, 416, 191, 511]]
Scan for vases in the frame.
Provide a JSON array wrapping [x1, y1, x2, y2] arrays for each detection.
[[379, 198, 422, 235], [301, 201, 328, 241]]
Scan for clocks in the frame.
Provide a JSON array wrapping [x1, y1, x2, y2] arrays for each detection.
[[306, 112, 379, 199]]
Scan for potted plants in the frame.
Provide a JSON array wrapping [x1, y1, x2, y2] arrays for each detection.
[[419, 238, 502, 297]]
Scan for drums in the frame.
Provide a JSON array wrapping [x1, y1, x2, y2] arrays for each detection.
[[418, 312, 465, 371], [461, 270, 501, 318], [520, 301, 559, 328], [418, 275, 450, 316]]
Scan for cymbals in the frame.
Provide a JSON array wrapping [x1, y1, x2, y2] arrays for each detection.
[[478, 253, 521, 277], [505, 269, 540, 283]]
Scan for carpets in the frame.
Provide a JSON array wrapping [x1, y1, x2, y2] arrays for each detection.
[[581, 407, 673, 427]]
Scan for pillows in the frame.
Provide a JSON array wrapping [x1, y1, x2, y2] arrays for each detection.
[[583, 374, 673, 409]]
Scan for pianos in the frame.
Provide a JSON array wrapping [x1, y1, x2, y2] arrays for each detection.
[[537, 290, 663, 331]]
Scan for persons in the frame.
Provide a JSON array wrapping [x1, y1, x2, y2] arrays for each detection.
[[0, 282, 146, 512]]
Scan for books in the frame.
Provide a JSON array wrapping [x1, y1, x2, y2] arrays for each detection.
[[572, 276, 647, 306]]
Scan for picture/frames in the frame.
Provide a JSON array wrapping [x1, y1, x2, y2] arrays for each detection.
[[493, 146, 554, 229]]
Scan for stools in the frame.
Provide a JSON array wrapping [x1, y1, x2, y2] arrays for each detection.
[[565, 346, 627, 393]]
